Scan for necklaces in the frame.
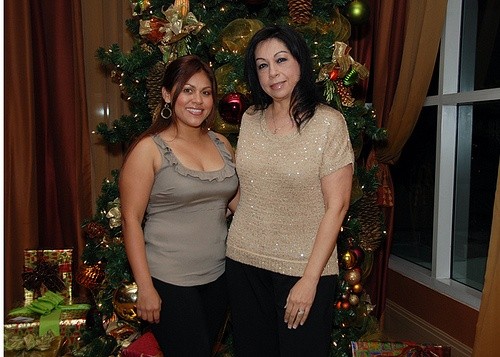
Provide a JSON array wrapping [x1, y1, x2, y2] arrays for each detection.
[[268, 105, 301, 135]]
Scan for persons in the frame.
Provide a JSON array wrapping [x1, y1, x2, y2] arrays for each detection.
[[224, 26, 356, 357], [117, 56, 241, 356]]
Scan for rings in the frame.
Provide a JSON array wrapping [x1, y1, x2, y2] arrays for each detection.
[[298, 310, 305, 314]]
